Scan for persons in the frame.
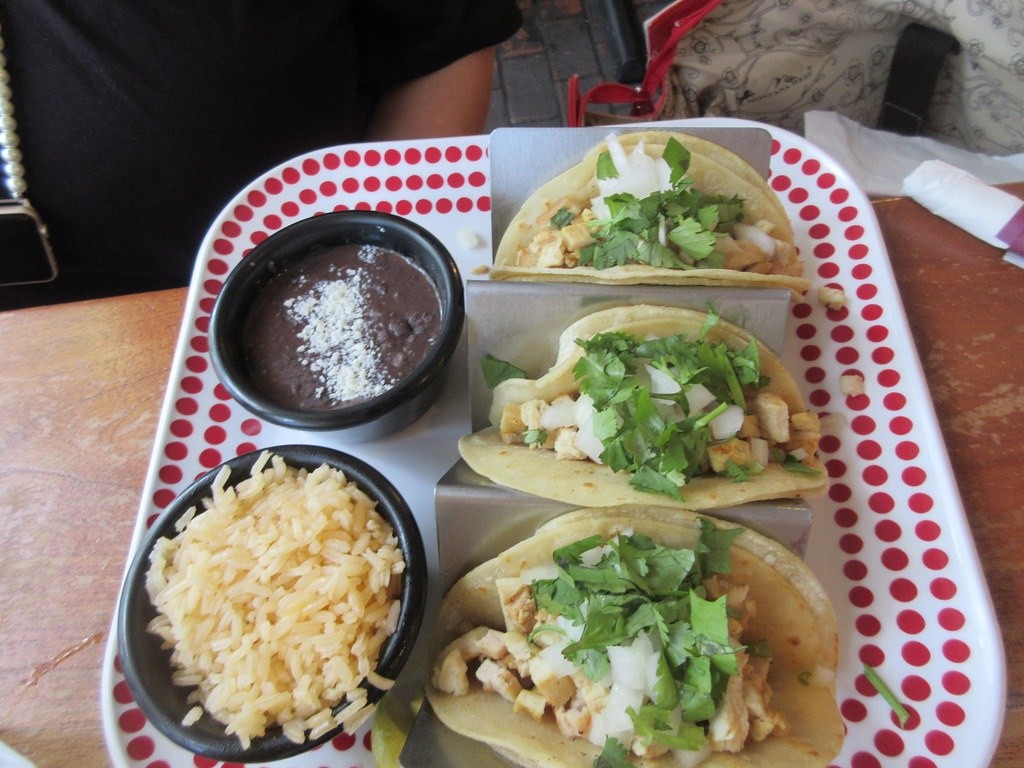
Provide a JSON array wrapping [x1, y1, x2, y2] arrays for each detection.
[[0, 0, 527, 318]]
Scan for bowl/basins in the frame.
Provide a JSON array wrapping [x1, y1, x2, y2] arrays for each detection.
[[117, 446, 428, 763], [207, 210, 465, 442]]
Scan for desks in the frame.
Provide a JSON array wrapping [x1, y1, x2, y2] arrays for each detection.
[[0, 184, 1024, 768]]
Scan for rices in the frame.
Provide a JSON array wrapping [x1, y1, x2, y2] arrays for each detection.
[[147, 451, 406, 745]]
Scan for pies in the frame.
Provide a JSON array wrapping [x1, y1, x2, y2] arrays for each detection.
[[494, 130, 814, 291], [427, 502, 849, 768], [457, 306, 831, 510]]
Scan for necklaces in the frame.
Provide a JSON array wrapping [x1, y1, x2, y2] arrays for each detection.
[[0, 22, 28, 201]]
